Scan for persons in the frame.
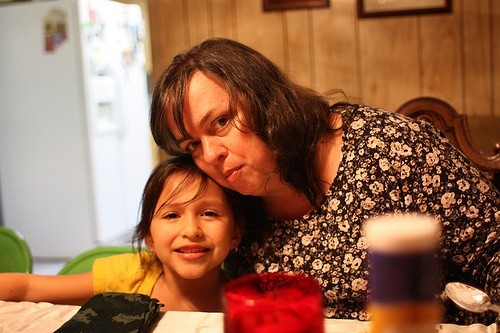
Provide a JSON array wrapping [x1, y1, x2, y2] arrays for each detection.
[[0, 156, 246, 312], [149, 38, 500, 333]]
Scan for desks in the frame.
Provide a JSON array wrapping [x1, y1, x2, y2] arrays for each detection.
[[0, 299, 497, 333]]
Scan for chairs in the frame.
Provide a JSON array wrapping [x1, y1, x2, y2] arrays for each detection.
[[0, 226, 33, 273], [395, 96, 500, 184]]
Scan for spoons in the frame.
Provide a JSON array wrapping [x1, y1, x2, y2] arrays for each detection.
[[446, 282, 500, 313]]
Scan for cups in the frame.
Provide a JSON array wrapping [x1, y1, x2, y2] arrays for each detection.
[[224, 273, 325, 333], [365, 213, 442, 333]]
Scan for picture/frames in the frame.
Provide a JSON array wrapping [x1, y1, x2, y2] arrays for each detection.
[[263, 0, 329, 11], [357, 0, 452, 18]]
